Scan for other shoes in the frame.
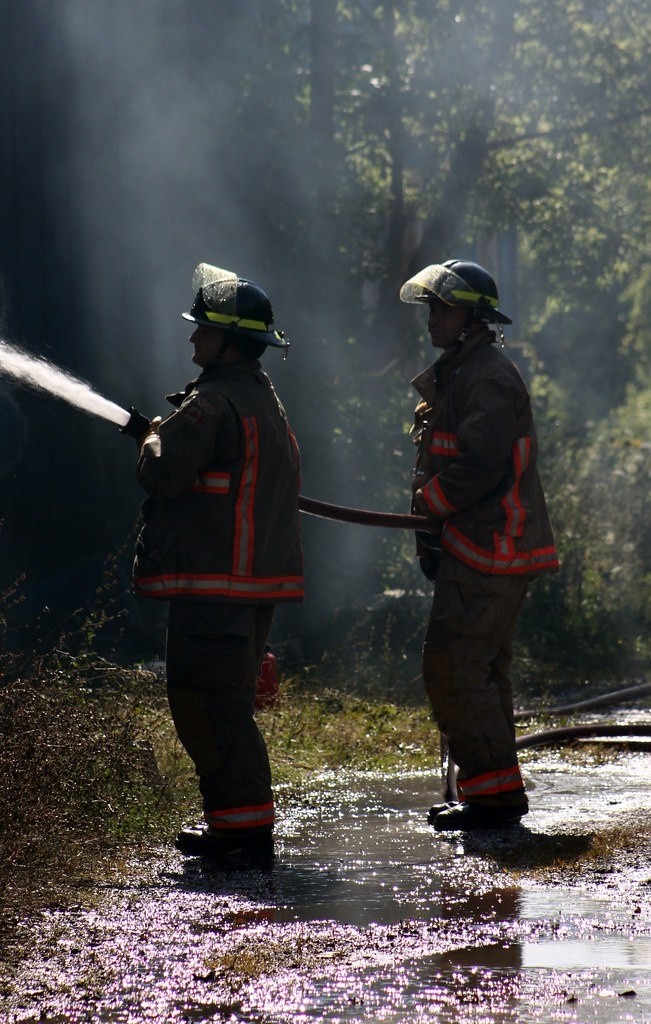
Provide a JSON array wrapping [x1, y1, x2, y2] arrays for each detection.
[[177, 827, 273, 857], [427, 799, 463, 823], [435, 796, 529, 829]]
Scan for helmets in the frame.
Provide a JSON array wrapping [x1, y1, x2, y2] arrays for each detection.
[[412, 259, 513, 325], [182, 278, 292, 349]]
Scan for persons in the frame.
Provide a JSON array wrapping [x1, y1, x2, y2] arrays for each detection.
[[131, 263, 299, 869], [399, 259, 559, 828]]
